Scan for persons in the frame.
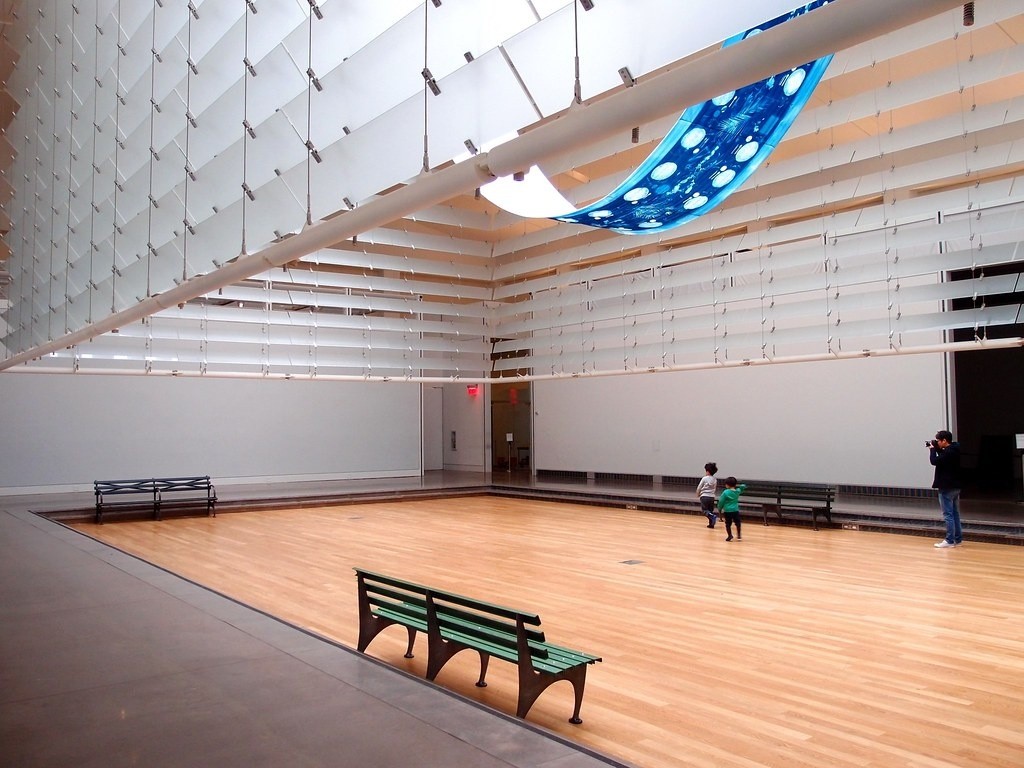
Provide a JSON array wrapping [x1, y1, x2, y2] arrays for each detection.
[[926, 430, 963, 548], [717, 477, 747, 542], [695, 463, 718, 528]]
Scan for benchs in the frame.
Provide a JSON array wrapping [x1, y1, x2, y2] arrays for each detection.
[[349, 567, 602, 726], [716, 480, 836, 531], [93, 475, 217, 524]]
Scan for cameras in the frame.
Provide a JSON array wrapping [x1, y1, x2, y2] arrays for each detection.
[[924, 439, 938, 447]]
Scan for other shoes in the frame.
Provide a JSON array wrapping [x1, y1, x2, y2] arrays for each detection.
[[737, 537, 741, 540], [712, 515, 716, 525], [707, 525, 714, 528], [954, 542, 963, 546], [726, 536, 733, 541], [934, 540, 955, 548]]
